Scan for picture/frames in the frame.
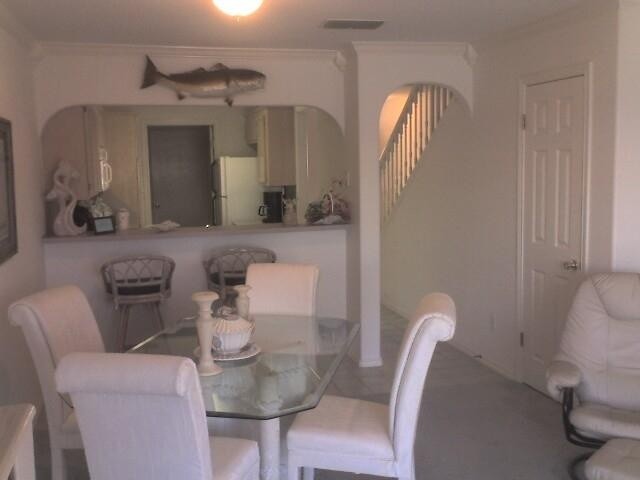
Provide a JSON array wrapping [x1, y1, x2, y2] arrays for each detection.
[[1, 114, 19, 267]]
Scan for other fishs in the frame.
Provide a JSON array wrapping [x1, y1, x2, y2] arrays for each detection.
[[139, 53, 266, 108]]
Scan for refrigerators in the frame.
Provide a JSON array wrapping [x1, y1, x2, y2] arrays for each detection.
[[211, 156, 264, 225]]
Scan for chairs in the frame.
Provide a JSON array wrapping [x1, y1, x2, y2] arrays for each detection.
[[202, 246, 274, 305], [246, 262, 318, 400], [584, 438, 640, 480], [8, 285, 106, 479], [100, 254, 177, 352], [287, 292, 457, 479], [55, 352, 260, 479], [545, 271, 640, 479]]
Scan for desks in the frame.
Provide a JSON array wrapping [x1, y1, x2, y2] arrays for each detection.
[[124, 314, 361, 480], [0, 402, 37, 479]]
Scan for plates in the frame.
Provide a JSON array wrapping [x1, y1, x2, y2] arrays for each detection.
[[192, 342, 262, 361]]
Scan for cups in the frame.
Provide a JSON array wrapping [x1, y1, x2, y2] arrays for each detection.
[[118, 209, 129, 231]]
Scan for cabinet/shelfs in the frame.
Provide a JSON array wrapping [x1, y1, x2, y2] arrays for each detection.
[[43, 106, 111, 200], [258, 107, 342, 191]]
